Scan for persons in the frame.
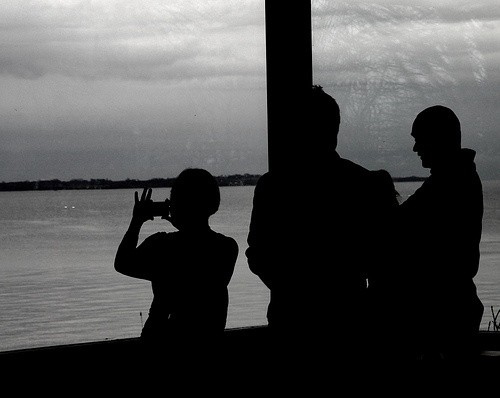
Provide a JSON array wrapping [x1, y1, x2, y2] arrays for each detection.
[[247, 84, 402, 398], [114, 168, 239, 398], [398, 105, 486, 398]]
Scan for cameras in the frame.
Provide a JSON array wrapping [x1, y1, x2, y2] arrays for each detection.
[[148, 201, 170, 216]]
